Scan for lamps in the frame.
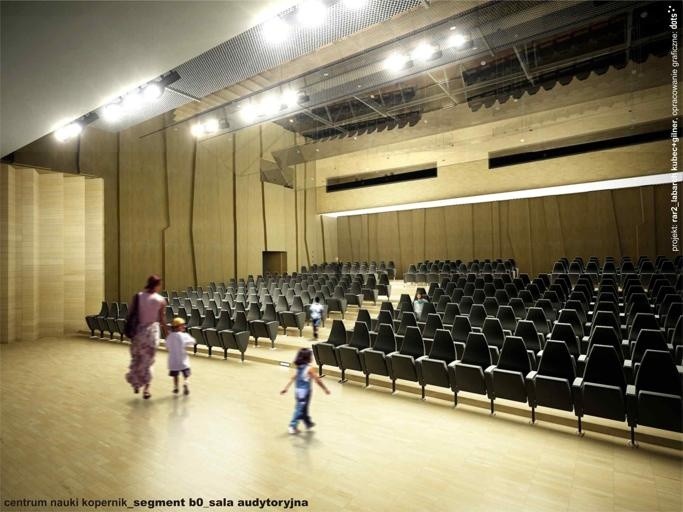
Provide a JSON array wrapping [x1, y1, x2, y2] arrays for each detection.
[[388, 28, 474, 76], [242, 75, 310, 118], [105, 71, 182, 125], [188, 109, 230, 136], [51, 113, 98, 146]]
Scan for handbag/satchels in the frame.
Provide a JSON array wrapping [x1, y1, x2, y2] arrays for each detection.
[[124, 315, 138, 338]]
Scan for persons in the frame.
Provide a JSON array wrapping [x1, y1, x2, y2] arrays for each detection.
[[309, 297, 324, 339], [166, 317, 197, 395], [280, 347, 331, 434], [413, 293, 429, 317], [125, 275, 168, 399]]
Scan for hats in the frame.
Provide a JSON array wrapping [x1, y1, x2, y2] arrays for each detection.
[[171, 317, 185, 327]]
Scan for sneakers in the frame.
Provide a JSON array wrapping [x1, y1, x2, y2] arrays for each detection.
[[135, 388, 150, 399], [172, 381, 188, 395], [289, 423, 314, 434]]
